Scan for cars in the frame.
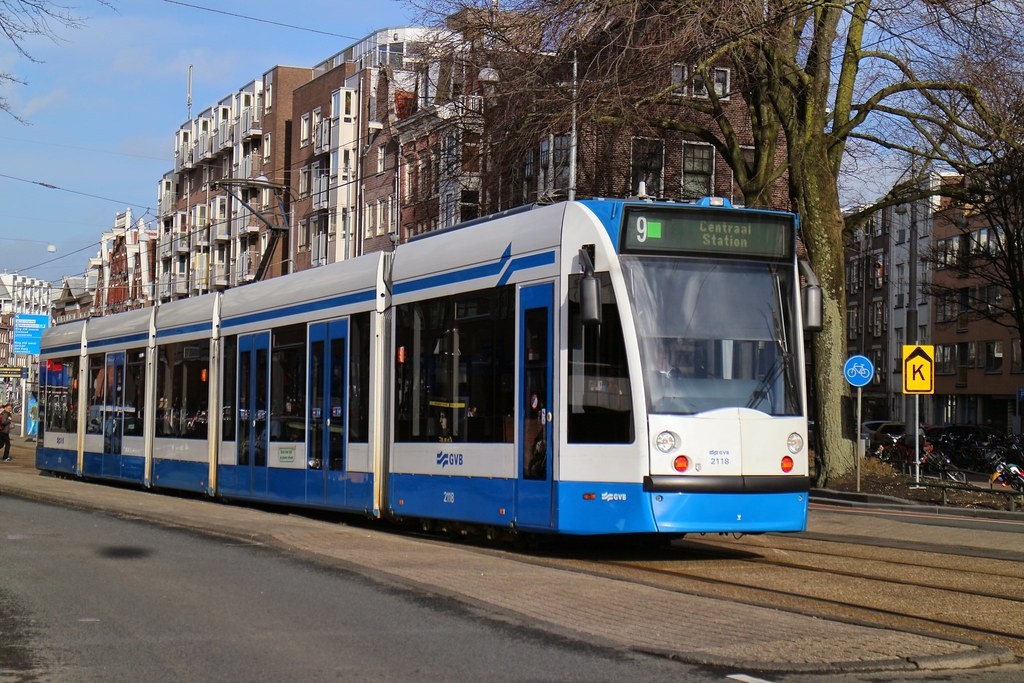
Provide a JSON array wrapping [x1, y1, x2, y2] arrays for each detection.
[[862, 420, 1003, 456]]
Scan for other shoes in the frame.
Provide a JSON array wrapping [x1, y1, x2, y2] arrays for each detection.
[[3, 458, 13, 462]]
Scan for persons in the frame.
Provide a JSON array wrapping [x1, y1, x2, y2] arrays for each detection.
[[644, 339, 688, 378], [0, 404, 16, 463]]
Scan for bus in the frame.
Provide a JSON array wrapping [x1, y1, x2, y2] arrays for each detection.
[[34, 180, 824, 558]]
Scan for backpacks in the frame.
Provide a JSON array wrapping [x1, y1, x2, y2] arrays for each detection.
[[0, 413, 6, 430]]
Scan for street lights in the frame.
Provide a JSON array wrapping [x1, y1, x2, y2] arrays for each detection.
[[475, 50, 578, 201], [893, 144, 920, 345]]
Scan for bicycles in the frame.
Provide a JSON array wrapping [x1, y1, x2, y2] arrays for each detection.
[[865, 427, 1024, 494]]
